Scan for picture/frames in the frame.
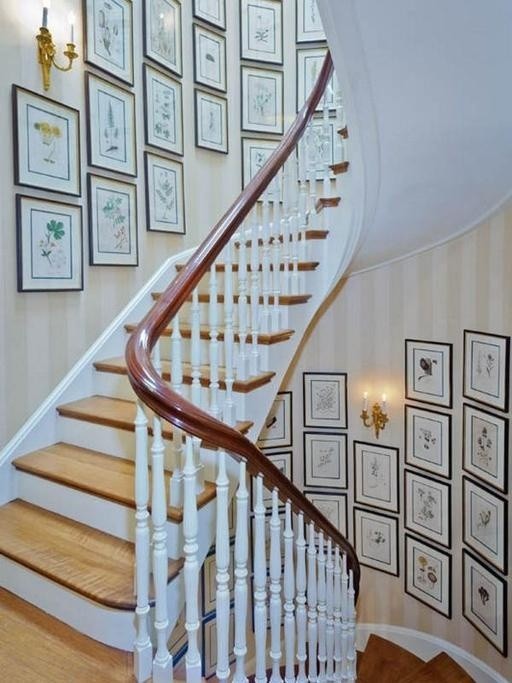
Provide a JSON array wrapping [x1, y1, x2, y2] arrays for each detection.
[[170, 514, 294, 683], [15, 193, 85, 293], [461, 475, 508, 575], [192, 1, 227, 32], [296, 46, 342, 117], [81, 70, 138, 177], [12, 83, 82, 199], [304, 431, 347, 487], [193, 88, 229, 155], [302, 371, 347, 428], [296, 1, 327, 44], [296, 125, 336, 182], [258, 390, 292, 450], [241, 137, 282, 203], [303, 490, 349, 549], [238, 1, 284, 65], [403, 467, 451, 550], [86, 172, 140, 268], [241, 65, 285, 136], [464, 329, 509, 413], [142, 62, 184, 158], [403, 532, 454, 620], [142, 149, 187, 235], [403, 336, 455, 410], [352, 441, 400, 513], [142, 1, 184, 78], [351, 508, 400, 576], [401, 404, 452, 481], [461, 405, 508, 494], [254, 449, 292, 511], [192, 23, 228, 94], [80, 1, 135, 88], [460, 547, 508, 657]]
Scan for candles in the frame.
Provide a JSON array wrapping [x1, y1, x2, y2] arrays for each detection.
[[68, 12, 78, 44], [362, 392, 371, 412], [40, 2, 51, 27], [380, 393, 388, 414]]
